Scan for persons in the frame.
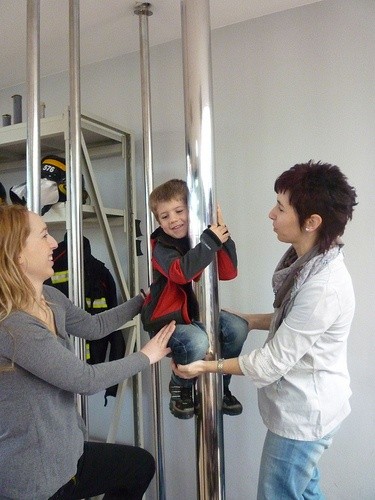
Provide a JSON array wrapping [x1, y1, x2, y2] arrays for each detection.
[[140, 180, 248, 420], [170, 159, 359, 500], [0, 204, 176, 500]]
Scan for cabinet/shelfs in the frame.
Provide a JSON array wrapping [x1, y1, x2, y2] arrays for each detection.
[[1, 104, 144, 449]]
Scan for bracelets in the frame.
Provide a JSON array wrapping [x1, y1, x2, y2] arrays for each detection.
[[216, 359, 227, 377], [141, 288, 146, 297]]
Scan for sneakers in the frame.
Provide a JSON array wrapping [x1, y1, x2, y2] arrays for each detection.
[[223, 389, 242, 415], [168, 378, 195, 419]]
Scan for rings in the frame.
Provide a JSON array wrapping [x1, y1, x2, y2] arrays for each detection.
[[184, 377, 186, 380]]
